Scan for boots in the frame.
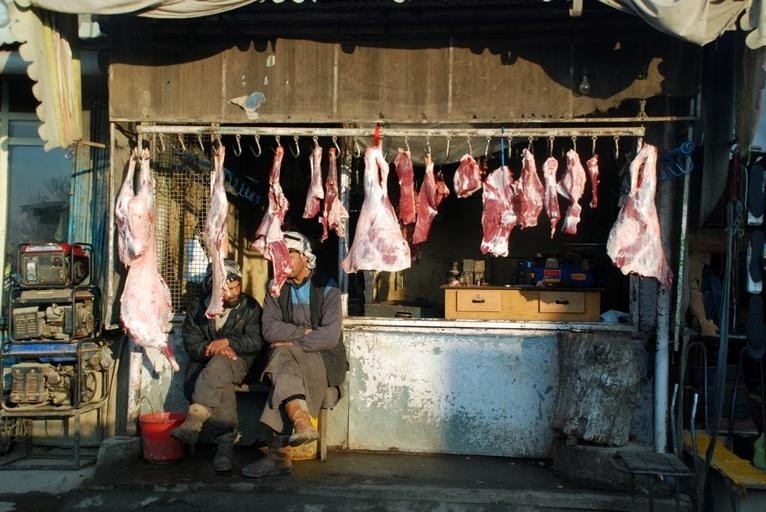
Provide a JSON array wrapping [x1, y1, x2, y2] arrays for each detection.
[[170, 403, 211, 446], [242, 399, 320, 477], [213, 432, 235, 471]]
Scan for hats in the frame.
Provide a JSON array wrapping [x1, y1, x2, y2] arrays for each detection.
[[282, 231, 311, 257], [202, 260, 241, 293]]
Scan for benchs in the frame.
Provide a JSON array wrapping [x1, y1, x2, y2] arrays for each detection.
[[236, 382, 346, 463], [680, 430, 766, 511]]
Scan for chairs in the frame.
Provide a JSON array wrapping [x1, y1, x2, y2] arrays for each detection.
[[608, 342, 712, 512]]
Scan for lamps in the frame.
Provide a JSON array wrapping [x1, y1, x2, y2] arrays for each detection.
[[578, 54, 594, 95]]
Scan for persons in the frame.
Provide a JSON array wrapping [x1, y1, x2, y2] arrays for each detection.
[[684, 227, 722, 336], [167, 256, 265, 475], [243, 232, 350, 479]]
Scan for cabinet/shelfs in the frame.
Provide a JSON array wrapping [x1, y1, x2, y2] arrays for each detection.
[[443, 286, 601, 321], [0, 241, 110, 470]]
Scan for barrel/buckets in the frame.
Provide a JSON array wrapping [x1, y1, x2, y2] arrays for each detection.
[[138, 396, 185, 461]]
